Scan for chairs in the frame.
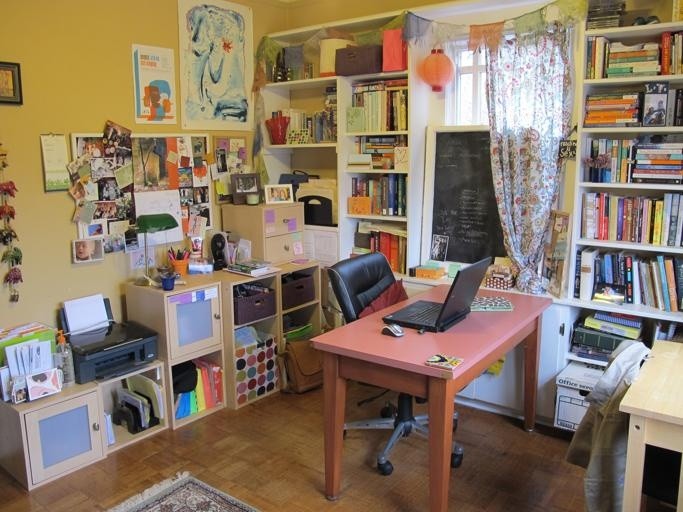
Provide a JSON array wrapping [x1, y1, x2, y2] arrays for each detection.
[[329, 251, 464, 475]]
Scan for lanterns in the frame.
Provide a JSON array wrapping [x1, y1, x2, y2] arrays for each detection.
[[419, 48, 454, 92]]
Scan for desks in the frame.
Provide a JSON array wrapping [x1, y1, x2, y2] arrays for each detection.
[[307, 285, 553, 512], [619, 338, 683, 512]]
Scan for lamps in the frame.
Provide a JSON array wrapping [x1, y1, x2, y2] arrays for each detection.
[[125, 211, 181, 289]]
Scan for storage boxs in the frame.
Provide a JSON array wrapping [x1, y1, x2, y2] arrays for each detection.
[[232, 284, 279, 327], [554, 360, 605, 434], [281, 275, 317, 313]]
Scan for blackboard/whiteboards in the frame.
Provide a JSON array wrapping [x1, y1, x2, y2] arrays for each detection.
[[418, 124, 508, 275]]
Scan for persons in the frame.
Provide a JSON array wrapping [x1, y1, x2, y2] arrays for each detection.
[[68, 181, 85, 200], [75, 240, 96, 261]]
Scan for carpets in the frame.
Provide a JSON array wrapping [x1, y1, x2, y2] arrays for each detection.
[[104, 471, 262, 512]]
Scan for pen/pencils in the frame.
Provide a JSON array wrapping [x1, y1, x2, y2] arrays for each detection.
[[167, 246, 192, 260]]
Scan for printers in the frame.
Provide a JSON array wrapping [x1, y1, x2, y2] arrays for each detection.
[[59, 297, 161, 385]]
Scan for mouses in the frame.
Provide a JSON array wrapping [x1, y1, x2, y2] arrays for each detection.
[[382, 324, 405, 337]]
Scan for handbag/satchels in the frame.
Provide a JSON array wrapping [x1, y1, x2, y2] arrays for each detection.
[[277, 341, 324, 394]]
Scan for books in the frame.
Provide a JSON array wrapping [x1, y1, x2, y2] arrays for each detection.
[[345, 79, 408, 275], [470, 295, 514, 311], [0, 322, 62, 404], [222, 260, 283, 278], [569, 0, 682, 363]]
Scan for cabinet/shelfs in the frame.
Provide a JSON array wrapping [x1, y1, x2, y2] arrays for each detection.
[[230, 274, 287, 411], [220, 203, 310, 266], [257, 9, 408, 276], [2, 378, 111, 490], [279, 261, 321, 369], [91, 359, 170, 457], [124, 275, 228, 431], [567, 0, 683, 322]]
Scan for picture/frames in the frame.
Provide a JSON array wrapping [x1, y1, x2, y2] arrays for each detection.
[[263, 184, 293, 204], [231, 173, 261, 194], [178, 0, 255, 131], [131, 44, 176, 125], [0, 61, 23, 104]]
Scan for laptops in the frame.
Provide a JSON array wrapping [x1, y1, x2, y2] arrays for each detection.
[[382, 256, 493, 333]]
